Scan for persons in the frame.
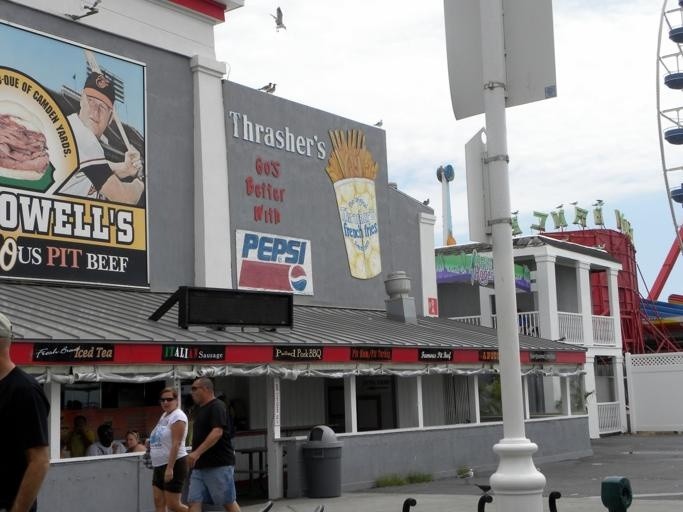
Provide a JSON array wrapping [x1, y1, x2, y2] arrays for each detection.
[[185, 378, 242, 512], [60, 71, 145, 207], [84, 424, 126, 456], [0, 312, 50, 512], [124, 430, 146, 453], [149, 387, 189, 512], [66, 415, 95, 457]]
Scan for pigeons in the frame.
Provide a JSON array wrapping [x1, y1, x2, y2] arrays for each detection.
[[270, 7, 286, 32], [474, 483, 491, 493], [423, 199, 429, 206], [512, 210, 519, 215], [456, 468, 474, 482], [570, 201, 578, 205], [596, 199, 603, 203], [259, 83, 277, 95], [374, 119, 383, 127], [556, 204, 563, 208]]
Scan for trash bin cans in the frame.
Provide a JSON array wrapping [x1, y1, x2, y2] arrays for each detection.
[[302, 425, 344, 498]]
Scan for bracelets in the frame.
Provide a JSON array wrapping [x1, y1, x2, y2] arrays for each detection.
[[137, 175, 144, 183]]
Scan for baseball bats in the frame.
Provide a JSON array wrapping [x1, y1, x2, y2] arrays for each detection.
[[82, 47, 130, 149]]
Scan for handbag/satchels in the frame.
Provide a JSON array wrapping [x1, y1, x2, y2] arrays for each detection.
[[142, 446, 154, 469]]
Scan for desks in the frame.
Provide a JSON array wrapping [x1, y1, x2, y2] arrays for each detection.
[[236, 445, 286, 493]]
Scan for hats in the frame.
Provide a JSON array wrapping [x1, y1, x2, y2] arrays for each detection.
[[83, 71, 116, 111], [0, 313, 13, 338]]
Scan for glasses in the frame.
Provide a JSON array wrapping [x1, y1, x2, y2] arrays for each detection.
[[191, 386, 204, 392], [158, 396, 176, 403]]
